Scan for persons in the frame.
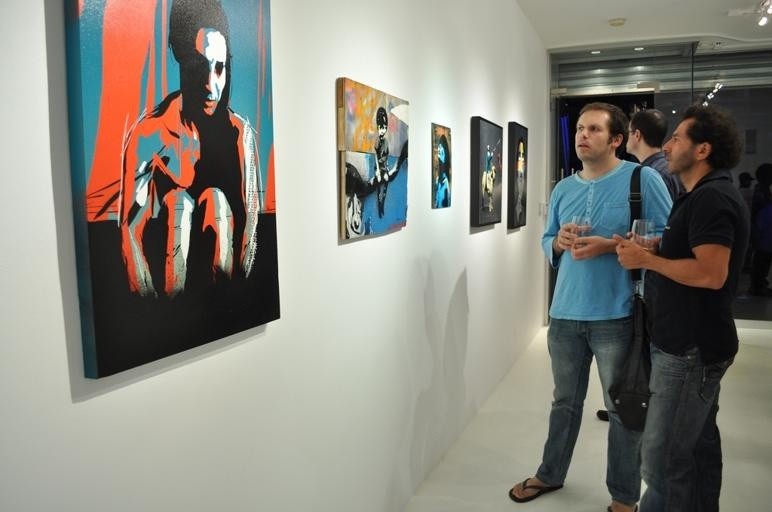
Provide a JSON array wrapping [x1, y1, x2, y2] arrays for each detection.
[[486, 145, 496, 172], [612, 101, 753, 512], [117, 0, 264, 301], [749, 163, 772, 296], [738, 172, 753, 273], [514, 138, 526, 225], [511, 102, 674, 512], [435, 134, 451, 208], [373, 106, 389, 218], [597, 108, 679, 421]]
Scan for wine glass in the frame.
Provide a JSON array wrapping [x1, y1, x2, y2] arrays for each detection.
[[570, 216, 592, 245]]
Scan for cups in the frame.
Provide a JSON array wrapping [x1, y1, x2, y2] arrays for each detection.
[[630, 218, 656, 287]]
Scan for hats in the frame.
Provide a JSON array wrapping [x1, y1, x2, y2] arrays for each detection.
[[738, 172, 755, 180]]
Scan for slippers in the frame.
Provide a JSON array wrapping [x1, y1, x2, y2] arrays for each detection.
[[509, 478, 565, 503]]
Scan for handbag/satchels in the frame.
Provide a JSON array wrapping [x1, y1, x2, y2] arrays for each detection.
[[608, 296, 650, 432]]
